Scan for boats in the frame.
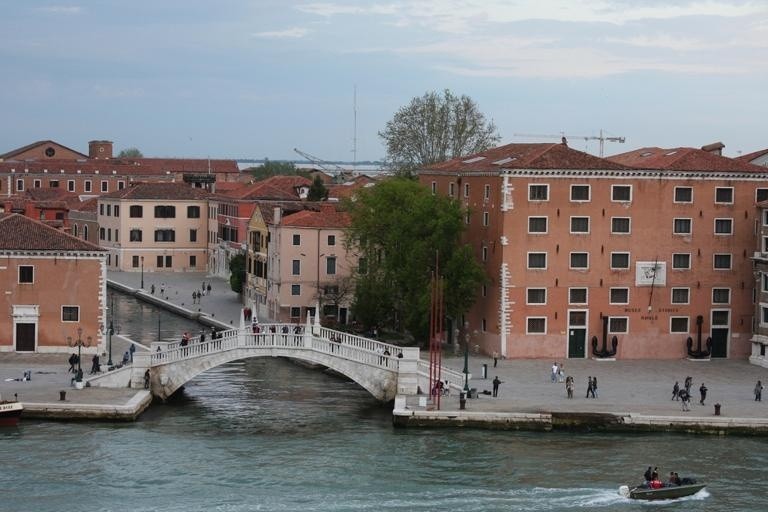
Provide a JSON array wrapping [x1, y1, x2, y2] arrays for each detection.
[[618, 479, 707, 504], [1, 393, 24, 427]]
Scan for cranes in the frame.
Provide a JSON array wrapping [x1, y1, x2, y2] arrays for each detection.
[[511, 126, 629, 161]]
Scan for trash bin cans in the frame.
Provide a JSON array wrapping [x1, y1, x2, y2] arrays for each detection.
[[481, 364, 488, 379], [460, 391, 467, 410]]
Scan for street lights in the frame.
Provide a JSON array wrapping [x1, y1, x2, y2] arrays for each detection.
[[139, 255, 145, 289], [98, 313, 122, 366], [109, 291, 114, 318], [66, 324, 92, 390], [453, 320, 481, 399]]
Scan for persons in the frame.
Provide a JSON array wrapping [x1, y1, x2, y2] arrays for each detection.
[[395, 350, 404, 368], [430, 379, 451, 397], [68, 351, 100, 385], [280, 324, 289, 346], [191, 290, 197, 304], [328, 333, 336, 353], [491, 375, 502, 397], [753, 380, 764, 401], [197, 290, 202, 305], [199, 328, 206, 353], [493, 349, 499, 368], [122, 351, 129, 365], [156, 345, 162, 359], [201, 281, 206, 296], [247, 307, 252, 321], [299, 324, 305, 346], [382, 347, 391, 367], [642, 465, 681, 489], [142, 368, 150, 390], [243, 306, 248, 321], [269, 324, 276, 344], [178, 332, 192, 358], [293, 323, 300, 347], [549, 361, 599, 400], [250, 317, 257, 324], [150, 284, 156, 295], [252, 322, 261, 345], [158, 282, 168, 296], [335, 336, 342, 355], [207, 282, 211, 296], [670, 372, 709, 412], [375, 345, 383, 365], [129, 343, 136, 362], [210, 326, 218, 348]]
